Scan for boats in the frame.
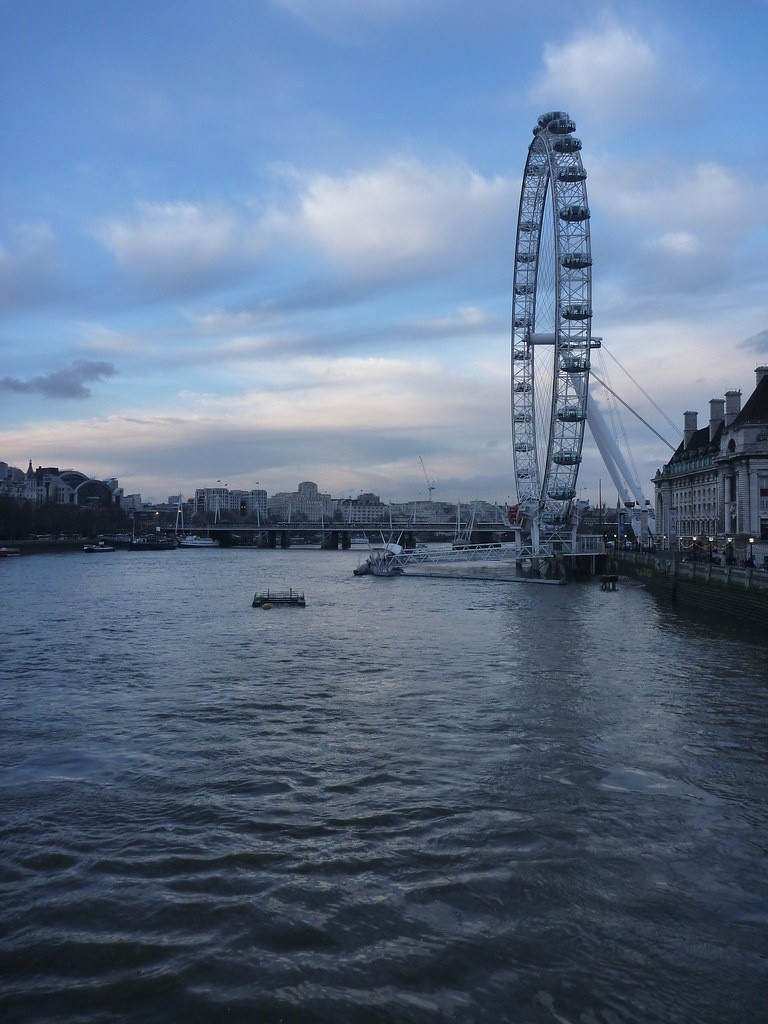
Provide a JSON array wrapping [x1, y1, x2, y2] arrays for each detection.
[[84, 544, 115, 552], [180, 535, 220, 548], [370, 564, 404, 577], [0, 547, 21, 557], [127, 534, 178, 551], [413, 544, 428, 553]]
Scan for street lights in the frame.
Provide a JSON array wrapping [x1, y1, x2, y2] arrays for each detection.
[[663, 536, 666, 551], [614, 534, 616, 548], [728, 536, 732, 563], [749, 538, 755, 567], [693, 536, 697, 560], [679, 536, 683, 552], [709, 537, 713, 562], [605, 534, 607, 549], [625, 534, 627, 549], [575, 488, 586, 504]]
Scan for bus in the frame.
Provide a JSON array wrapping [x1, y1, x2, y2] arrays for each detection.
[[60, 534, 81, 540], [36, 535, 50, 540]]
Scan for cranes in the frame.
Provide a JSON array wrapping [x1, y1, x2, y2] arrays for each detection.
[[419, 456, 437, 501]]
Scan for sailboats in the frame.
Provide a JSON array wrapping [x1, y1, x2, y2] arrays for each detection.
[[452, 498, 477, 549]]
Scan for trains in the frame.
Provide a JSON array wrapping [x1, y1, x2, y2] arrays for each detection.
[[277, 522, 506, 529]]
